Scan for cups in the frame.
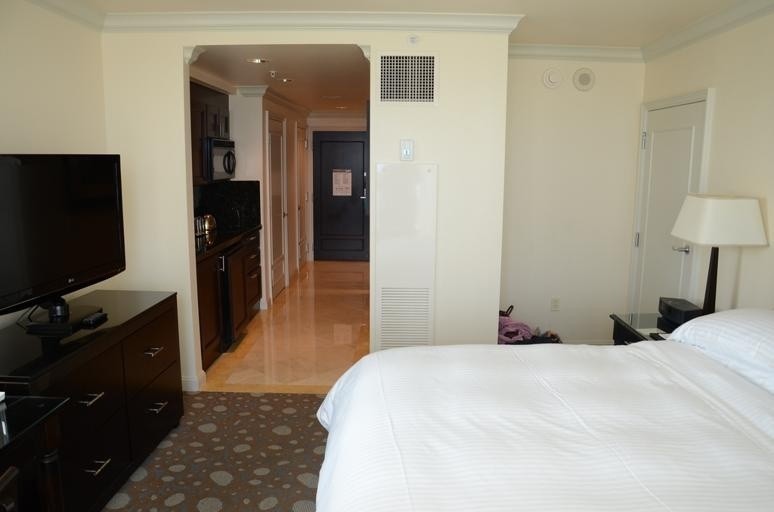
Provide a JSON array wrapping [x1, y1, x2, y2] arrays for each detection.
[[194, 236, 204, 252], [193, 216, 203, 234]]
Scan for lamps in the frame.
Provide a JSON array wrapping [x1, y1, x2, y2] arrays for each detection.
[[670, 193, 770, 313]]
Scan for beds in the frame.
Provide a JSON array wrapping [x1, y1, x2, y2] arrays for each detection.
[[312, 302, 772, 512]]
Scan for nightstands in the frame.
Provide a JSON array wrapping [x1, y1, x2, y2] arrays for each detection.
[[608, 313, 677, 344]]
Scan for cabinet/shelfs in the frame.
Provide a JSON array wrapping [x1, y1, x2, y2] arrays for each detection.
[[195, 250, 227, 370], [210, 89, 230, 138], [0, 288, 186, 512], [229, 86, 286, 314], [295, 104, 311, 275], [226, 227, 264, 349], [190, 81, 210, 187]]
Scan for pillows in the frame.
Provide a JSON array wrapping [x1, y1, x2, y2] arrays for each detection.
[[665, 304, 773, 393]]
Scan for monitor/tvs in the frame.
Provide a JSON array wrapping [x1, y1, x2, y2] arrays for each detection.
[[0, 154, 126, 337]]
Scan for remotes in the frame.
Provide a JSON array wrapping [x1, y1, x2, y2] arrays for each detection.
[[83, 312, 107, 325]]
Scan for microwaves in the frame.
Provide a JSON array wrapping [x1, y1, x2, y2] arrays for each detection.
[[202, 137, 236, 180]]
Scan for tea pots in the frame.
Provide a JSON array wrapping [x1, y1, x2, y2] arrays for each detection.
[[204, 230, 214, 246], [203, 214, 217, 230]]
